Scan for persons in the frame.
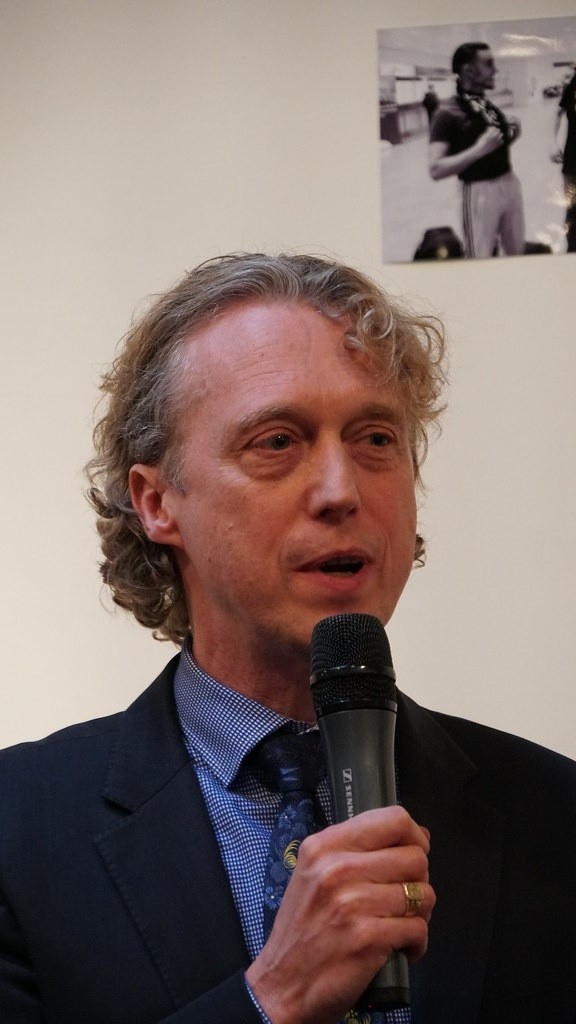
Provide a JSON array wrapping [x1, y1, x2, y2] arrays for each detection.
[[427, 39, 527, 257], [420, 83, 441, 125], [0, 253, 576, 1024]]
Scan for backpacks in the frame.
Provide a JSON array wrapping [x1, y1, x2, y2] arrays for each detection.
[[413, 226, 465, 260]]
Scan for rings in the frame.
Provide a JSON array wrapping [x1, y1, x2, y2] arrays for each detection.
[[402, 881, 424, 919]]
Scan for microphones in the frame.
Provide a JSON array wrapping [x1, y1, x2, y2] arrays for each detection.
[[306, 609, 411, 1013]]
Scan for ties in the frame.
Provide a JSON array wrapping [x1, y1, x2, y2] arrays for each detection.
[[248, 730, 329, 946]]
